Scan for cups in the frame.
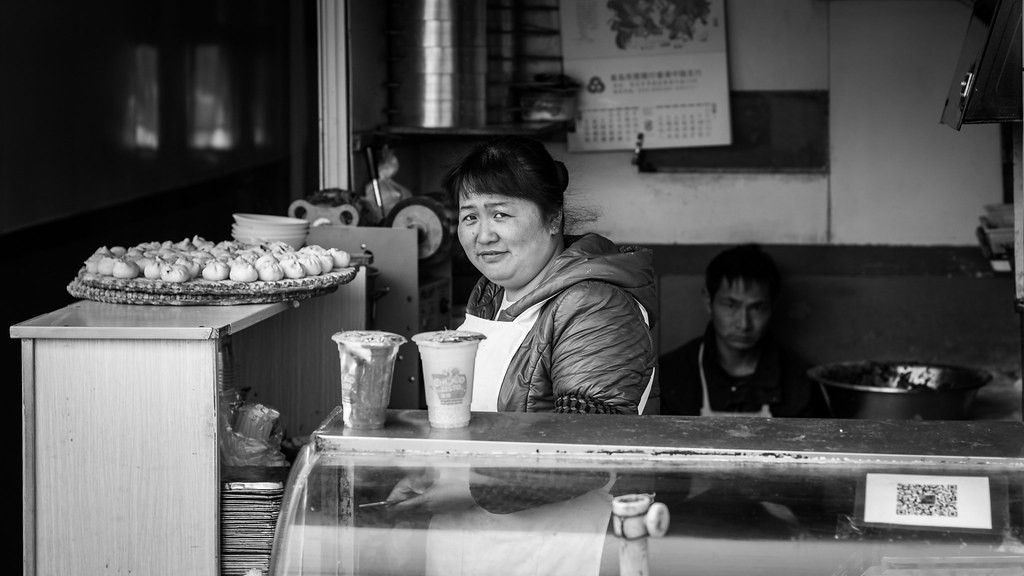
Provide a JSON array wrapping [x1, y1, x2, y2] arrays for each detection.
[[331, 330, 408, 431], [218, 345, 240, 421], [412, 331, 483, 429]]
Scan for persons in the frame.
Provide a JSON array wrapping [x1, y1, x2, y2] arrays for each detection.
[[656, 245, 828, 541], [384, 136, 660, 576]]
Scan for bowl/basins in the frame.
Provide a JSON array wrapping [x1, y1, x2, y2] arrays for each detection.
[[230, 213, 311, 251], [808, 360, 994, 421]]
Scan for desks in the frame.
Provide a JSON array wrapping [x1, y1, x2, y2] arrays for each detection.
[[7, 265, 366, 576]]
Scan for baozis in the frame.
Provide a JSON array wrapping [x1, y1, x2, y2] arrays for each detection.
[[83, 234, 351, 282]]
